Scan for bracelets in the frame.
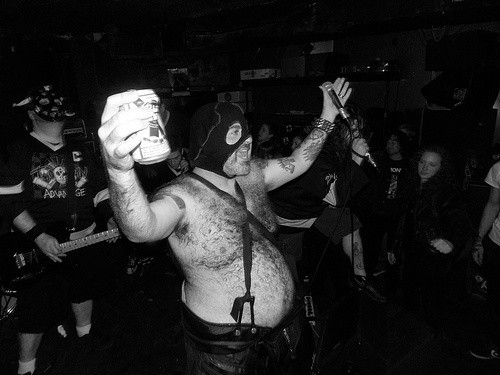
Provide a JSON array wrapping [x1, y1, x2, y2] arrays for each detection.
[[314, 117, 336, 135], [24, 222, 42, 242]]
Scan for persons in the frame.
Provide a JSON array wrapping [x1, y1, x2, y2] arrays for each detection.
[[98, 76, 353, 375], [258, 90, 500, 361], [99, 84, 189, 280], [0, 83, 118, 375]]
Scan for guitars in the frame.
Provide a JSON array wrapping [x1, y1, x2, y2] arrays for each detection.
[[0, 227, 122, 286]]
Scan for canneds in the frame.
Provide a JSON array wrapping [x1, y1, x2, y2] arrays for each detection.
[[119, 89, 171, 165]]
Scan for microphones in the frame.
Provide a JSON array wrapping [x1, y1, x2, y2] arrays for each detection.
[[322, 81, 352, 124], [365, 151, 377, 167]]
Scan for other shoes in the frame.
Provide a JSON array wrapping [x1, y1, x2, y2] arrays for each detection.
[[468, 337, 500, 360], [73, 325, 114, 352], [365, 280, 392, 303]]
